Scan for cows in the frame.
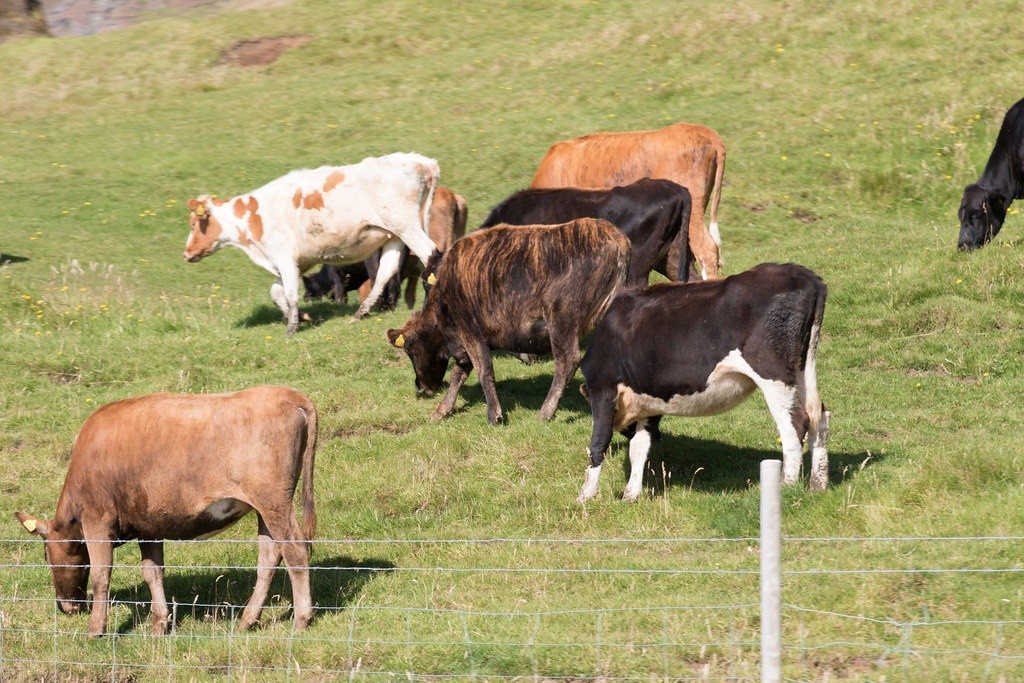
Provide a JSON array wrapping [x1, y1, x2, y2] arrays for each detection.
[[183, 151, 467, 334], [14, 385, 318, 638], [388, 124, 829, 502], [957, 97, 1024, 250]]
